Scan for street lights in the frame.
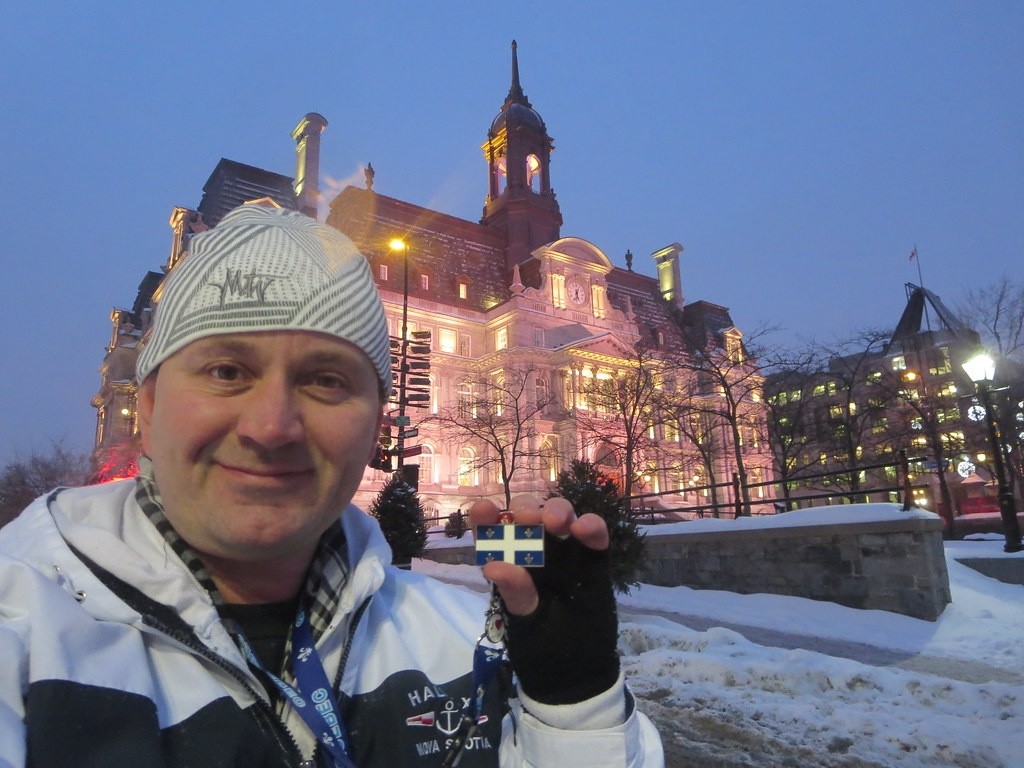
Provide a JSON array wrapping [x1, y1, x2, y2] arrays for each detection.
[[385, 239, 409, 481], [958, 353, 1023, 553]]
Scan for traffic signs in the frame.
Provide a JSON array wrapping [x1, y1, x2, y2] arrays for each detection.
[[403, 430, 419, 438]]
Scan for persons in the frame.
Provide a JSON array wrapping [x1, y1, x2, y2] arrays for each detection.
[[0, 207, 665, 768]]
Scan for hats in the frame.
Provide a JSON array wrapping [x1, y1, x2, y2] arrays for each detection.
[[135, 204, 390, 403]]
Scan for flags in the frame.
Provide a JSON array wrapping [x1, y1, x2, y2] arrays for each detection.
[[909, 248, 916, 261]]
